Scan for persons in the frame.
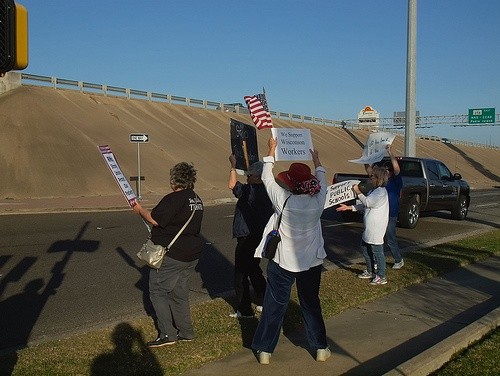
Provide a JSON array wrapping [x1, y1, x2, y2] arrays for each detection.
[[133, 161, 205, 348], [336, 169, 389, 285], [252, 137, 332, 365], [228, 153, 275, 318], [360, 144, 404, 269]]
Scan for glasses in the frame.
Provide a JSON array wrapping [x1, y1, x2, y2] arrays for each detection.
[[247, 175, 252, 177]]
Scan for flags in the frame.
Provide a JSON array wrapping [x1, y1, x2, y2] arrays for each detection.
[[244, 93, 273, 130]]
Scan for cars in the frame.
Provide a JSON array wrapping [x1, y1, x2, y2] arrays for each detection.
[[215, 103, 243, 112]]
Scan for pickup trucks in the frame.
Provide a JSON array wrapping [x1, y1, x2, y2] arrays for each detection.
[[332, 156, 471, 229]]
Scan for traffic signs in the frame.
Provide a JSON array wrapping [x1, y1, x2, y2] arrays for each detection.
[[468, 108, 495, 123], [129, 133, 149, 143]]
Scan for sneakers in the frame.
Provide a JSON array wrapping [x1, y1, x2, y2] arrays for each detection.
[[147, 334, 175, 348], [358, 270, 376, 278], [316, 345, 331, 361], [256, 349, 271, 364], [177, 335, 194, 341], [370, 274, 387, 285]]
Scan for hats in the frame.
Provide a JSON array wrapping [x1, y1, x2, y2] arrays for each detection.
[[246, 161, 264, 177], [278, 163, 320, 195]]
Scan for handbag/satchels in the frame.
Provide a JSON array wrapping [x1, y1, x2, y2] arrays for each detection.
[[136, 240, 166, 269], [261, 230, 281, 260]]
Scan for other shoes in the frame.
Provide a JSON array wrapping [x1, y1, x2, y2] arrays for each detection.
[[229, 311, 253, 318], [256, 306, 263, 313], [392, 259, 404, 269]]
